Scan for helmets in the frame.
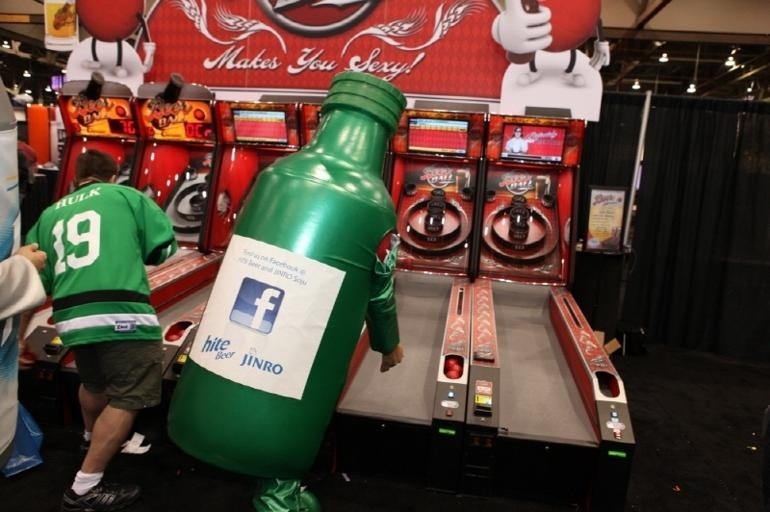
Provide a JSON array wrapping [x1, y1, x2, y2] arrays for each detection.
[[401, 197, 472, 254], [482, 204, 559, 263], [493, 207, 549, 250], [406, 200, 462, 242], [173, 182, 206, 223]]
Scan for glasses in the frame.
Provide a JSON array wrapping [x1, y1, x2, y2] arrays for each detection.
[[630, 52, 736, 94], [2, 39, 53, 95]]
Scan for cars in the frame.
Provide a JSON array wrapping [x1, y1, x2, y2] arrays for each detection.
[[196, 184, 209, 193], [462, 189, 472, 201], [535, 179, 546, 200], [190, 194, 207, 212], [510, 195, 530, 240], [542, 195, 554, 207], [485, 190, 496, 202], [405, 184, 416, 195], [425, 189, 445, 233], [455, 172, 466, 195]]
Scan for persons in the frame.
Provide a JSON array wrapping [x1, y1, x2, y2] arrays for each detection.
[[0, 240, 49, 317], [503, 125, 529, 156], [16, 123, 38, 183], [21, 149, 179, 510]]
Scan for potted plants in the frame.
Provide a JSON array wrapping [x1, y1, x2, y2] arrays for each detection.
[[581, 184, 628, 253]]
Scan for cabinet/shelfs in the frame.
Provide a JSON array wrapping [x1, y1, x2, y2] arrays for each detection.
[[157, 70, 407, 475], [72, 72, 105, 129], [152, 73, 185, 130]]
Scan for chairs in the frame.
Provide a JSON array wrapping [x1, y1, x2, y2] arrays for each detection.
[[78, 434, 90, 450], [62, 482, 139, 512]]
